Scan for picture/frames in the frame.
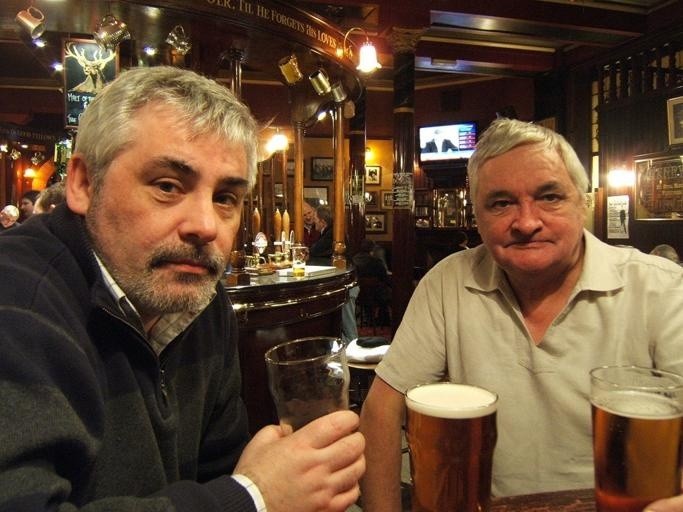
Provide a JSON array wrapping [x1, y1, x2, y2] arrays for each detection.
[[601, 95, 682, 245], [257, 152, 394, 237]]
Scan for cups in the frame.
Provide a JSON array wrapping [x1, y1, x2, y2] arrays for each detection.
[[404, 381, 499, 512], [590, 364, 682, 511], [265, 339, 349, 438], [292, 247, 307, 276]]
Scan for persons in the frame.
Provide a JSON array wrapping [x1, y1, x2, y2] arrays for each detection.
[[649, 244, 680, 263], [1, 65, 367, 512], [19, 191, 40, 216], [421, 129, 458, 151], [33, 182, 66, 214], [303, 201, 322, 245], [357, 112, 683, 512], [311, 207, 334, 257]]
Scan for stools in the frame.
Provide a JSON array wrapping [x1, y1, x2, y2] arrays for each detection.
[[345, 359, 382, 411]]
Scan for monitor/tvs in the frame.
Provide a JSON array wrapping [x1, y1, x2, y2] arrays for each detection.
[[417, 120, 480, 169]]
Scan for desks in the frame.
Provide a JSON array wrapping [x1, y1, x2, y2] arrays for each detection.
[[497, 487, 596, 512]]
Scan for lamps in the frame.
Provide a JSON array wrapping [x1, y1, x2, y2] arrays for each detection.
[[341, 24, 381, 74]]
